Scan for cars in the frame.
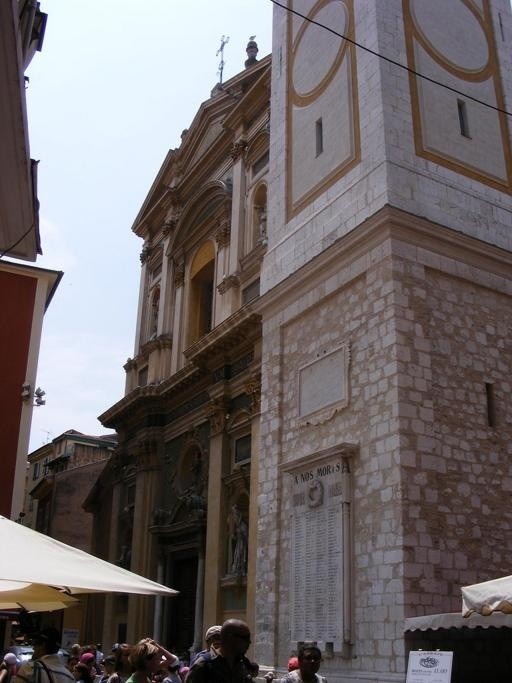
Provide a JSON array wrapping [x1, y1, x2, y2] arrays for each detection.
[[9, 645, 68, 665]]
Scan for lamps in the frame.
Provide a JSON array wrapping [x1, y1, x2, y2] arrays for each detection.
[[20, 381, 45, 408]]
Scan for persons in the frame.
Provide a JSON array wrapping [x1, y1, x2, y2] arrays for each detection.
[[0, 617, 327, 683]]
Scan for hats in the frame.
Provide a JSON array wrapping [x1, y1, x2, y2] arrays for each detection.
[[4, 653, 17, 664], [287, 657, 299, 671], [80, 653, 94, 663], [162, 654, 179, 668], [205, 626, 222, 640]]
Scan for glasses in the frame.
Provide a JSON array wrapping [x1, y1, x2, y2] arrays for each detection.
[[305, 656, 320, 661]]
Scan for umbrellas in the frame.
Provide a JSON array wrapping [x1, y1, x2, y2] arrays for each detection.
[[0, 582, 80, 617], [1, 514, 182, 598]]
